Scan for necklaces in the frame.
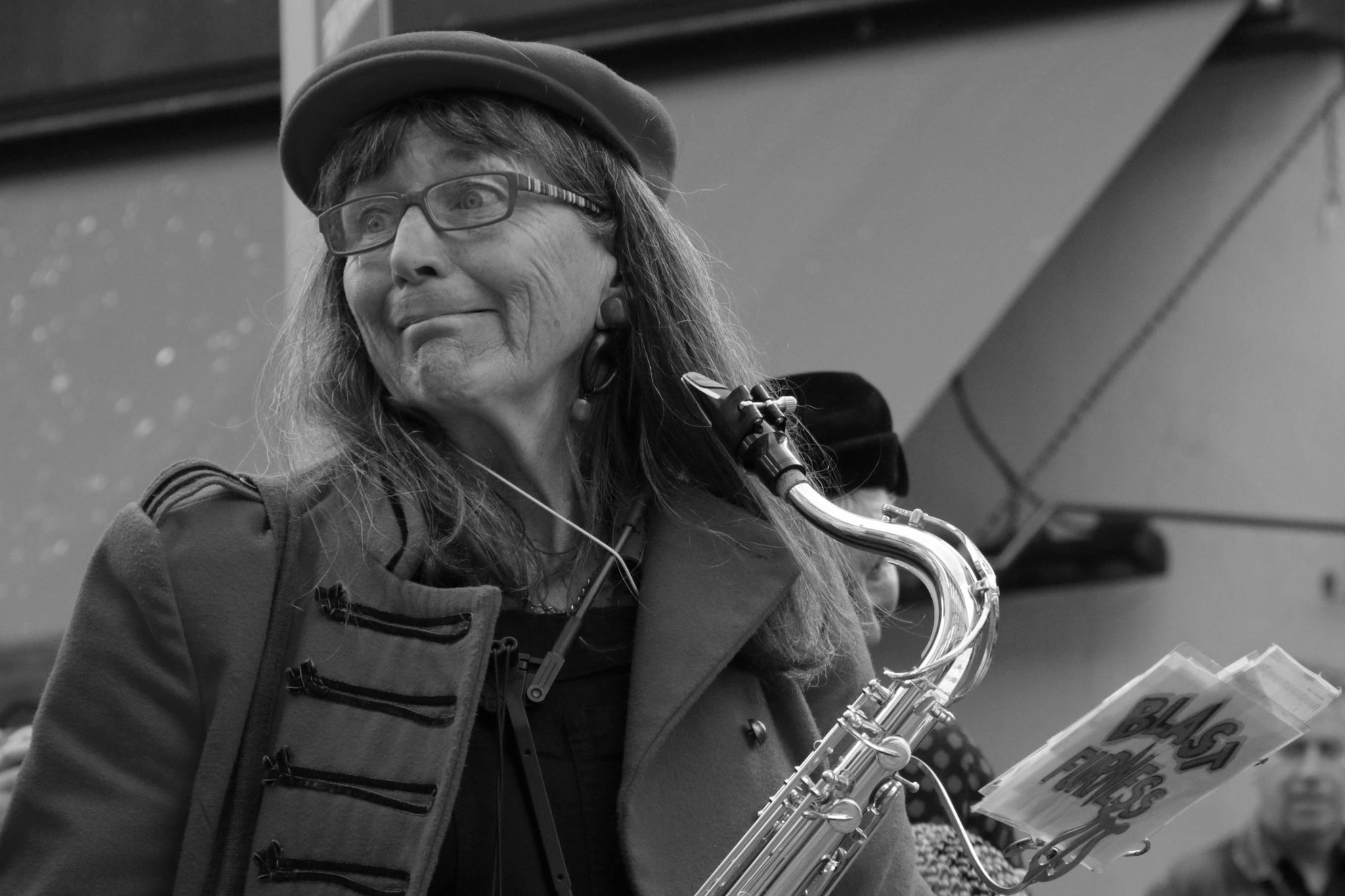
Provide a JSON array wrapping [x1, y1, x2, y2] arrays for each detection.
[[520, 562, 599, 619]]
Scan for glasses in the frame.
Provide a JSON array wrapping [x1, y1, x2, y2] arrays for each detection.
[[314, 170, 607, 255]]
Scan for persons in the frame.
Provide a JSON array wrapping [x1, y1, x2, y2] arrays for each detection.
[[1141, 667, 1345, 896], [0, 32, 935, 894], [747, 372, 1024, 870]]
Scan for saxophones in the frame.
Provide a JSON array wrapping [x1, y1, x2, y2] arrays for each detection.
[[680, 371, 1000, 895]]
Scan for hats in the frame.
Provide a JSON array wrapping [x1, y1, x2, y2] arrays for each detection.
[[276, 31, 677, 206], [763, 370, 909, 502]]
